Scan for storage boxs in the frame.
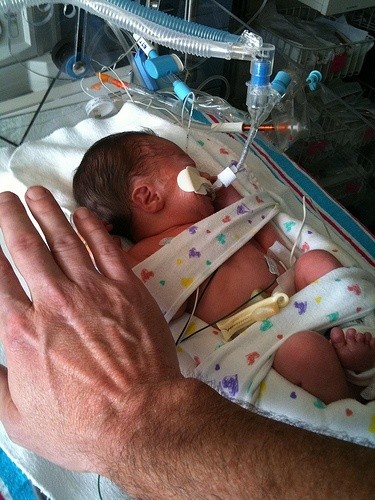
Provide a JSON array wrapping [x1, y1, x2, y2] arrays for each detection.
[[246, 6, 375, 208]]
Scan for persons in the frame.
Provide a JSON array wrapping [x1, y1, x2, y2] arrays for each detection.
[[73, 128, 375, 405], [1, 185, 375, 499]]
